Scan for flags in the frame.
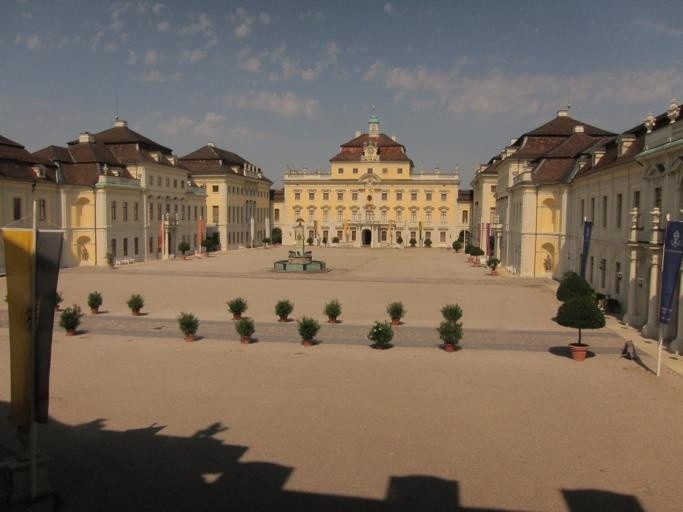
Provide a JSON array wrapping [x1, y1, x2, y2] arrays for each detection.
[[476, 222, 491, 256], [657, 221, 682, 325], [580, 217, 595, 280], [1, 228, 65, 428], [156, 215, 423, 248]]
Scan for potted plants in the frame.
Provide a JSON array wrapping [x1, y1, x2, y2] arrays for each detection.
[[557, 295, 604, 361], [436, 320, 465, 353], [296, 315, 320, 348], [440, 301, 464, 321], [452, 240, 500, 276], [59, 304, 82, 336], [178, 240, 192, 261], [365, 319, 395, 350], [88, 293, 102, 314], [322, 298, 343, 323], [274, 298, 296, 323], [126, 292, 146, 315], [386, 301, 407, 326], [54, 293, 63, 312], [227, 296, 248, 322], [233, 317, 257, 344], [177, 311, 200, 342]]
